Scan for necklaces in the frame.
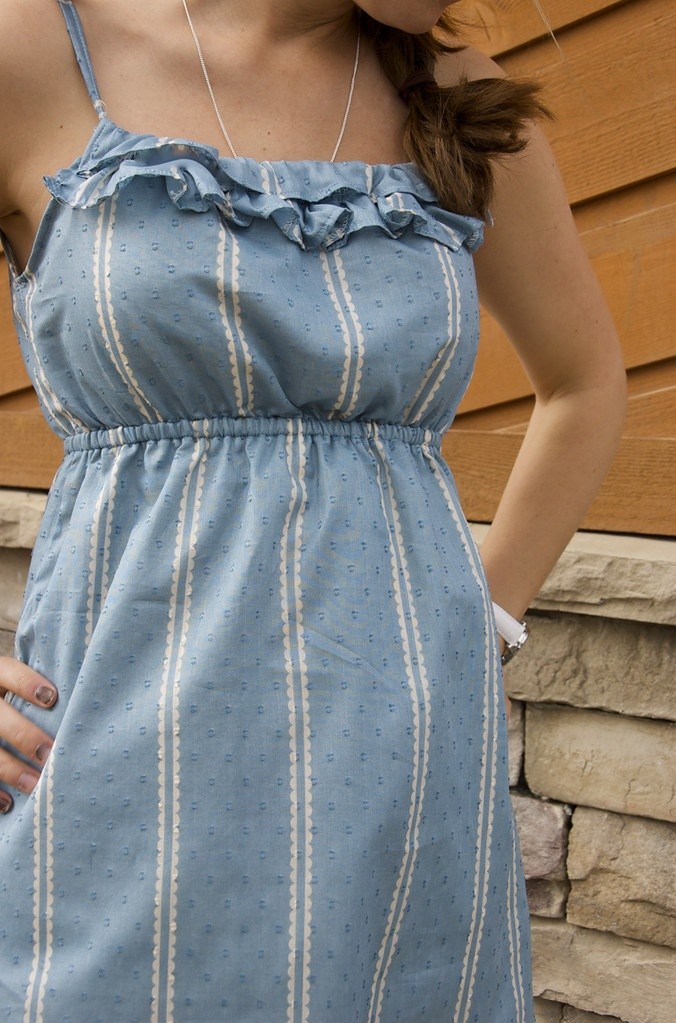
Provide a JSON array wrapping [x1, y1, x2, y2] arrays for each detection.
[[182, 0, 360, 163]]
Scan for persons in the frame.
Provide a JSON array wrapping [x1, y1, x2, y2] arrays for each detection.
[[0, 0, 627, 1023]]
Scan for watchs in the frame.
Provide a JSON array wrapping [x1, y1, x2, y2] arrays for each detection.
[[492, 601, 529, 666]]
